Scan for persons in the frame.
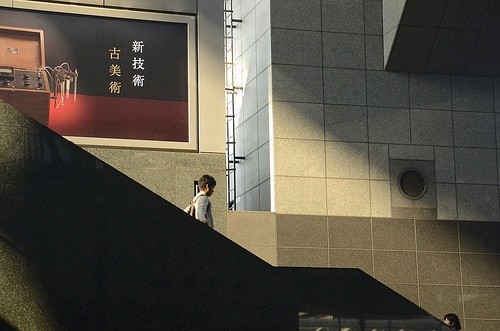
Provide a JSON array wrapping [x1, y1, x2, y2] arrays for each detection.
[[182, 174, 216, 230], [442, 313, 461, 331]]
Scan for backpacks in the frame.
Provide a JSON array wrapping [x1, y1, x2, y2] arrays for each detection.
[[183, 194, 210, 218]]
